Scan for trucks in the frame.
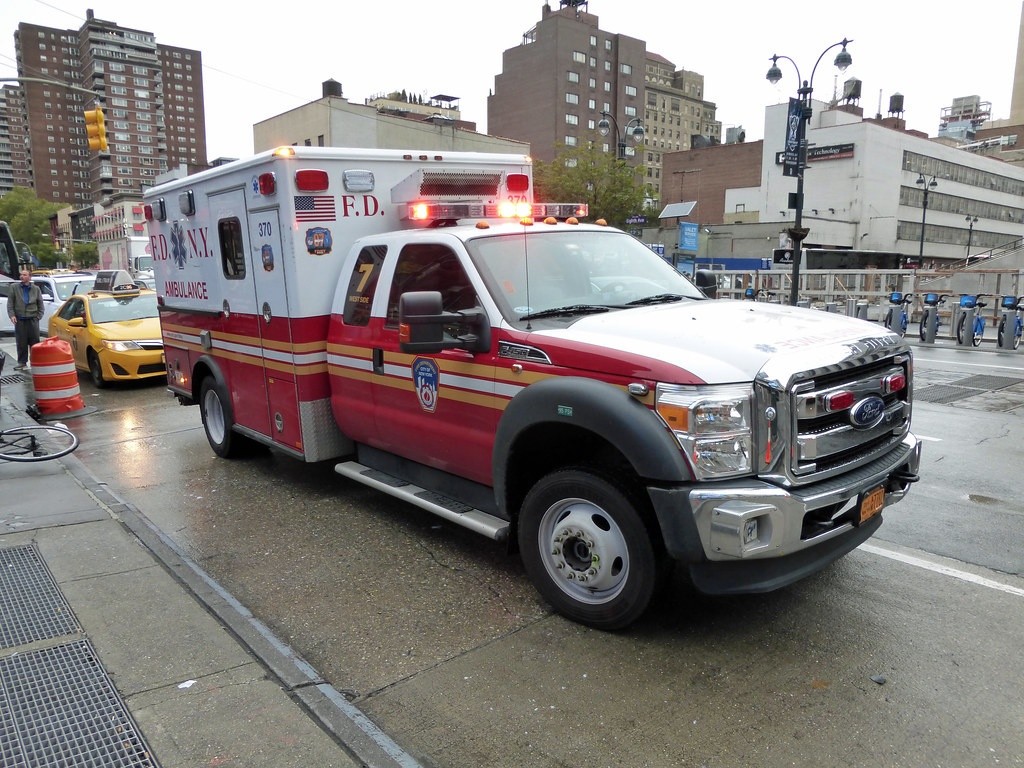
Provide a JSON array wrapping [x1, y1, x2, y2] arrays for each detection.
[[98, 236, 156, 289]]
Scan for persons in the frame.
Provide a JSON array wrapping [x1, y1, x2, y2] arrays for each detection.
[[683, 271, 695, 282], [8, 270, 45, 371], [546, 243, 603, 308]]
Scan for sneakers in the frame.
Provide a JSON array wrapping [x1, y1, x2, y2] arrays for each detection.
[[13, 364, 27, 370]]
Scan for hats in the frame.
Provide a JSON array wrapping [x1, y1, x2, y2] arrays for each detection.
[[440, 247, 457, 269]]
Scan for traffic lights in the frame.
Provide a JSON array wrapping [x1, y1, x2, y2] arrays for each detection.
[[84, 107, 107, 152]]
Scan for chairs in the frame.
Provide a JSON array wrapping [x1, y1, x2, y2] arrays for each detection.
[[94, 305, 112, 322]]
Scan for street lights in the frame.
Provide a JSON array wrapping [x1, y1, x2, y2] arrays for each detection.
[[965, 214, 978, 266], [766, 38, 854, 305], [598, 110, 645, 161], [104, 215, 121, 221], [42, 234, 61, 239], [916, 173, 938, 269], [15, 241, 31, 255]]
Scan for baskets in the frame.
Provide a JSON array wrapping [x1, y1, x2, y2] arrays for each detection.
[[924, 293, 938, 304], [959, 295, 976, 307], [1001, 295, 1017, 308], [889, 293, 901, 303]]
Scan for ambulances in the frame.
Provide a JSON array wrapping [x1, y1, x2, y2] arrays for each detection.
[[143, 145, 922, 632]]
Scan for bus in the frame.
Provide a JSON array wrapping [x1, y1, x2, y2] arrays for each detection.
[[771, 248, 907, 295], [0, 220, 30, 280]]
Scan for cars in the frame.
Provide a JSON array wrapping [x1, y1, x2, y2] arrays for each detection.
[[0, 279, 52, 331], [133, 278, 156, 291], [31, 276, 118, 332], [590, 277, 669, 293], [48, 270, 168, 389]]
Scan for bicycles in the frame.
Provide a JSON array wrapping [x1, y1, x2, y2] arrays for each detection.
[[884, 292, 913, 339], [957, 294, 993, 347], [0, 426, 80, 461], [919, 293, 954, 341], [998, 295, 1024, 350]]
